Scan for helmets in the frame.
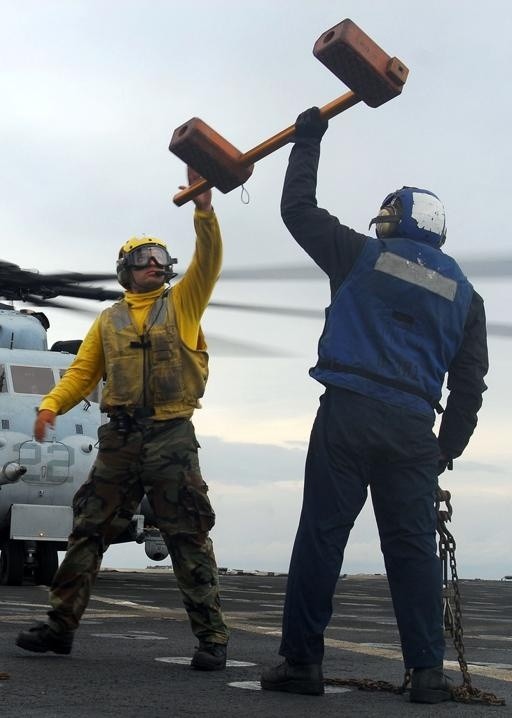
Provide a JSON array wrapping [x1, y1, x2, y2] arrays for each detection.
[[117, 234, 177, 288]]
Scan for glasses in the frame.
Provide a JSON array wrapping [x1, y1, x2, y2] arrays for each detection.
[[133, 248, 170, 267]]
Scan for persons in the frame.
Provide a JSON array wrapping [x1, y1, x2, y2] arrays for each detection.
[[12, 147, 239, 671], [262, 104, 489, 703]]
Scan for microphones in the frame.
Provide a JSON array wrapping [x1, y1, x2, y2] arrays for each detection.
[[156, 271, 177, 280]]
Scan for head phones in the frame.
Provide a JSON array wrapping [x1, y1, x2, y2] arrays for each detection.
[[116, 246, 173, 287]]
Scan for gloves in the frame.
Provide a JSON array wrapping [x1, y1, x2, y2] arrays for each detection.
[[296, 107, 328, 142]]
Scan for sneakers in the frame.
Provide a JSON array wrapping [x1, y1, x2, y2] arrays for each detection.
[[192, 641, 227, 669], [260, 662, 324, 695], [410, 671, 455, 703], [16, 624, 72, 654]]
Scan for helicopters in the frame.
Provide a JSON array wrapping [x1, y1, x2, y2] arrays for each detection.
[[0, 246, 512, 586]]
